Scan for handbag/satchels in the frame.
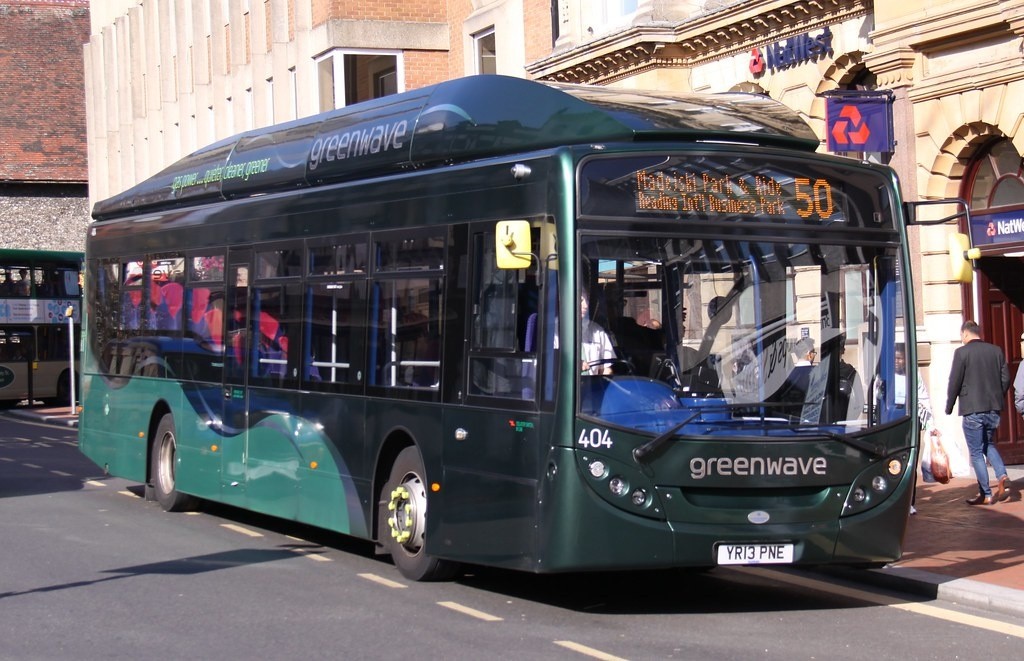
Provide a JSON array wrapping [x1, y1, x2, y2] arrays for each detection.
[[921, 430, 955, 484]]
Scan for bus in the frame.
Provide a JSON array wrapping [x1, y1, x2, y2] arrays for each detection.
[[80, 76, 921, 593], [0, 245, 137, 409]]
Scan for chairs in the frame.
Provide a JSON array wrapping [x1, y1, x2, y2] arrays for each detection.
[[119, 274, 322, 380]]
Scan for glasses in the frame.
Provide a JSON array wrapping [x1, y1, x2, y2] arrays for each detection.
[[811, 352, 817, 355]]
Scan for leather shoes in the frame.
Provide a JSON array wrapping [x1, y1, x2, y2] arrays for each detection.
[[994, 476, 1014, 501], [966, 492, 994, 505]]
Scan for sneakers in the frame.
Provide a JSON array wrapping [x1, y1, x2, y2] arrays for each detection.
[[909, 505, 916, 515]]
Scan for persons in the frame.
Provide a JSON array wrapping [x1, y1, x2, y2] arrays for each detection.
[[4, 268, 31, 296], [945, 320, 1011, 504], [732, 334, 864, 422], [643, 319, 661, 330], [1014, 333, 1024, 418], [553, 286, 617, 377], [872, 342, 937, 514]]
